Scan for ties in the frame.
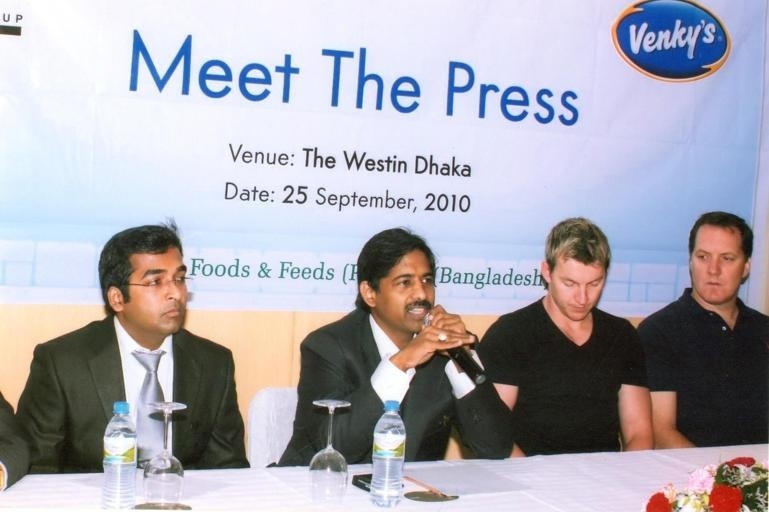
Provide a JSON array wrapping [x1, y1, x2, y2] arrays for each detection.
[[131, 348, 168, 472]]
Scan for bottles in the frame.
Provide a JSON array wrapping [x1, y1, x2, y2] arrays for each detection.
[[372, 401, 406, 509], [104, 402, 136, 509]]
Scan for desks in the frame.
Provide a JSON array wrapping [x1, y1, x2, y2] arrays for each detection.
[[0, 443, 768, 512]]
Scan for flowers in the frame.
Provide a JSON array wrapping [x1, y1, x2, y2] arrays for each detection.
[[643, 457, 768, 512]]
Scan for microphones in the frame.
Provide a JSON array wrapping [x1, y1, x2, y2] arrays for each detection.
[[423, 312, 487, 386]]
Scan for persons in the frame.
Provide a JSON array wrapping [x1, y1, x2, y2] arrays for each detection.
[[479, 218, 655, 458], [636, 212, 769, 449], [0, 391, 30, 491], [17, 226, 251, 473], [275, 227, 514, 466]]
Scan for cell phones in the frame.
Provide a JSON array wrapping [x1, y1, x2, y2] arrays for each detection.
[[352, 473, 404, 493]]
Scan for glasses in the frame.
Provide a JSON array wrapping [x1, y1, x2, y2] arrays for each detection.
[[124, 274, 192, 294]]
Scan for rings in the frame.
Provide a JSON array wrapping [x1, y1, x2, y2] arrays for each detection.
[[438, 333, 447, 342]]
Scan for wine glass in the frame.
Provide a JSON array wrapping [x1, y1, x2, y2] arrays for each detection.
[[144, 402, 186, 502], [309, 399, 351, 502]]
